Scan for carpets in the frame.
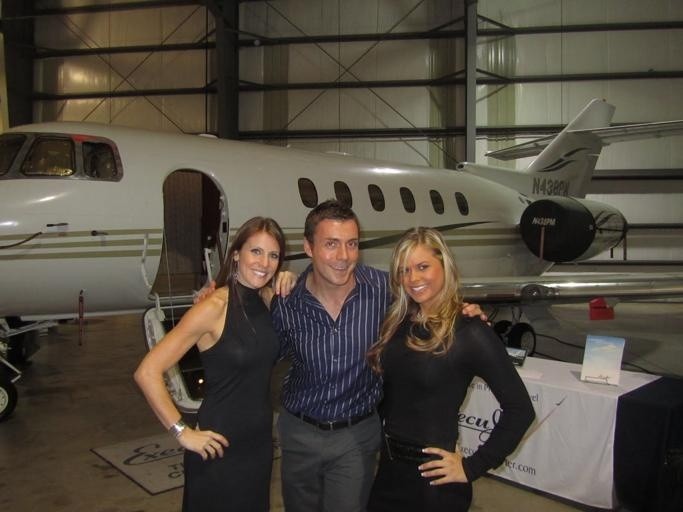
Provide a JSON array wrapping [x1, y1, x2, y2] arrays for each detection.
[[88, 411, 282, 497]]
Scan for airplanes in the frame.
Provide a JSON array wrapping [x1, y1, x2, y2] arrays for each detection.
[[0, 96, 683, 422]]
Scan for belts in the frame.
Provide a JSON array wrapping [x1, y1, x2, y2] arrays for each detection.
[[295, 411, 373, 430], [383, 438, 429, 462]]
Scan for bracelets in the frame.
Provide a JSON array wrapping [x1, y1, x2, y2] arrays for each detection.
[[166, 417, 187, 439]]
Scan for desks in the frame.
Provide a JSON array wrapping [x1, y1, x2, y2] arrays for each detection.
[[455, 349, 682, 511]]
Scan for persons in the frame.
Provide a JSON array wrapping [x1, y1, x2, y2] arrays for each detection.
[[130, 215, 301, 512], [364, 225, 537, 511], [3, 315, 32, 368], [191, 199, 494, 511]]
